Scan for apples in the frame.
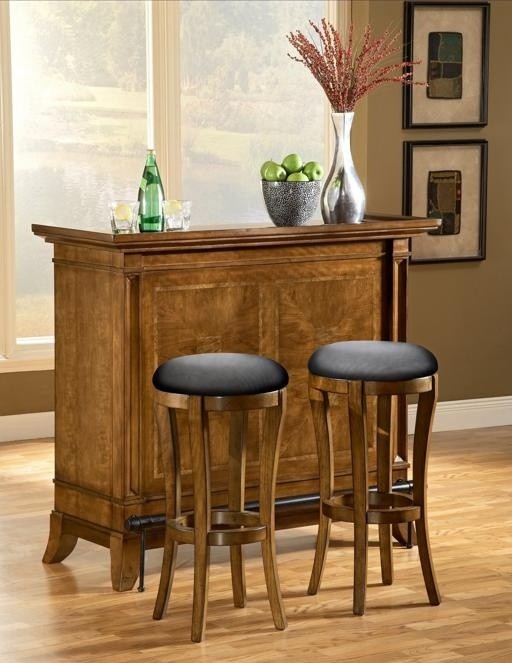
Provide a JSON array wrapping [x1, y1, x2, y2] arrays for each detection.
[[261, 153, 325, 181]]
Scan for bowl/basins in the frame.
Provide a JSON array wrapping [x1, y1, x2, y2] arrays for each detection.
[[261, 179, 322, 227]]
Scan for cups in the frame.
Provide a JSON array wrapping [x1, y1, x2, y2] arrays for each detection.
[[161, 200, 191, 232], [108, 199, 140, 234]]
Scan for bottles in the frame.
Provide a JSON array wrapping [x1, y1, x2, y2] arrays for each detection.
[[137, 148, 165, 233]]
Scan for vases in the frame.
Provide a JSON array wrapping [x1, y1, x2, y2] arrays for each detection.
[[320, 113, 366, 223]]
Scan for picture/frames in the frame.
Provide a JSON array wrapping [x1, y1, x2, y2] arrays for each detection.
[[403, 1, 490, 265]]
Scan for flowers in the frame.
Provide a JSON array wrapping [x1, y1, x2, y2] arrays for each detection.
[[285, 14, 432, 113]]
